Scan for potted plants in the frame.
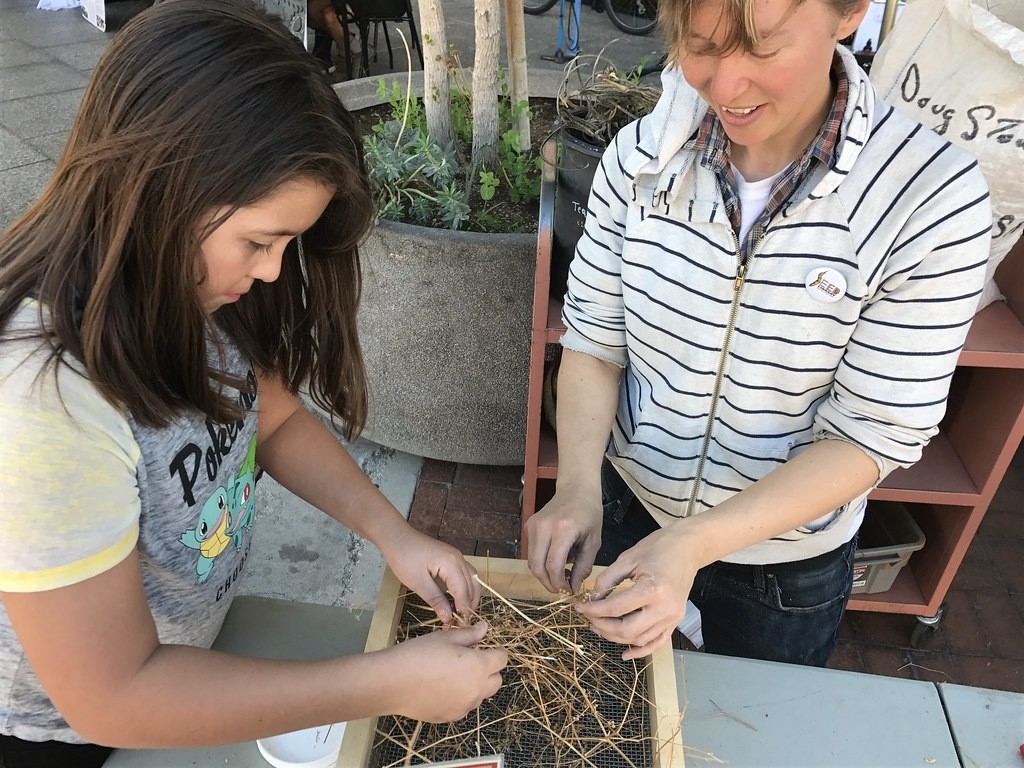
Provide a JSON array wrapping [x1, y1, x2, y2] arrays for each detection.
[[555, 36, 671, 255]]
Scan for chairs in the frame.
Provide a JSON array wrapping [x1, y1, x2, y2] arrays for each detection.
[[332, 0, 424, 81]]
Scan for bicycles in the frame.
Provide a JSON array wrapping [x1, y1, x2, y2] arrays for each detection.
[[524, 0, 658, 37]]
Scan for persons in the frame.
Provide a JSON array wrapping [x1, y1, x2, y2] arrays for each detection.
[[523, 0, 994, 666], [0, 0, 509, 768], [308, 0, 410, 75]]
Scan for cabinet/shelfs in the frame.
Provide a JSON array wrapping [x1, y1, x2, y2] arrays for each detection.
[[521, 141, 1024, 653]]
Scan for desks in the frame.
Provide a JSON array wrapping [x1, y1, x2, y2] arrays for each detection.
[[101, 593, 1024, 768]]
